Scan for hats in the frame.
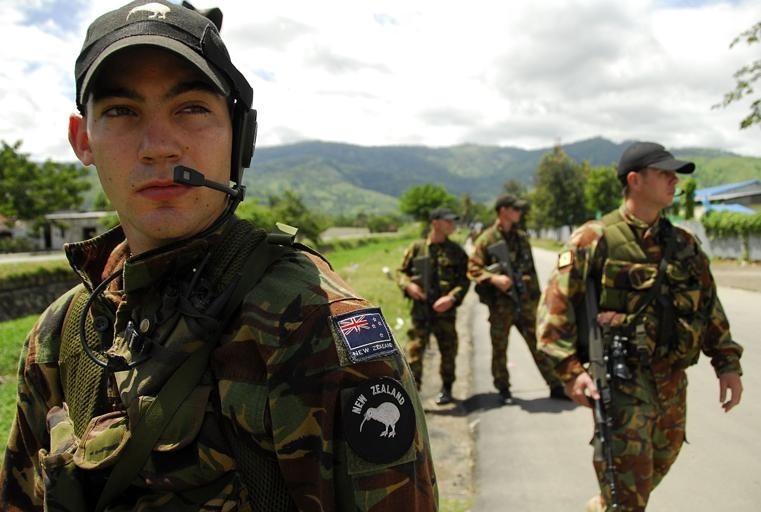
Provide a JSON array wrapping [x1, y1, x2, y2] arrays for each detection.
[[616, 141, 697, 178], [71, 0, 234, 106], [428, 206, 460, 223], [493, 193, 528, 210]]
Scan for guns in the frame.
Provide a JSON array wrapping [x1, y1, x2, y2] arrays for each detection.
[[486, 241, 533, 342], [411, 255, 431, 346], [575, 282, 632, 512]]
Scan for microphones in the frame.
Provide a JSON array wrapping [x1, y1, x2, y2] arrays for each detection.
[[174, 165, 247, 201]]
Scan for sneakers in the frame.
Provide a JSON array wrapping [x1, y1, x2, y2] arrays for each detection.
[[547, 386, 575, 403], [433, 381, 453, 406], [496, 389, 515, 405]]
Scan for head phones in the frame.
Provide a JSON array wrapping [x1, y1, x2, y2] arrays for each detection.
[[230, 93, 257, 187]]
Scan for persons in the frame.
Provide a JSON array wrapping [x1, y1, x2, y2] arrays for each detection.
[[0, 1, 440, 511], [465, 193, 574, 405], [396, 208, 470, 405], [470, 215, 486, 246], [535, 140, 744, 512]]
